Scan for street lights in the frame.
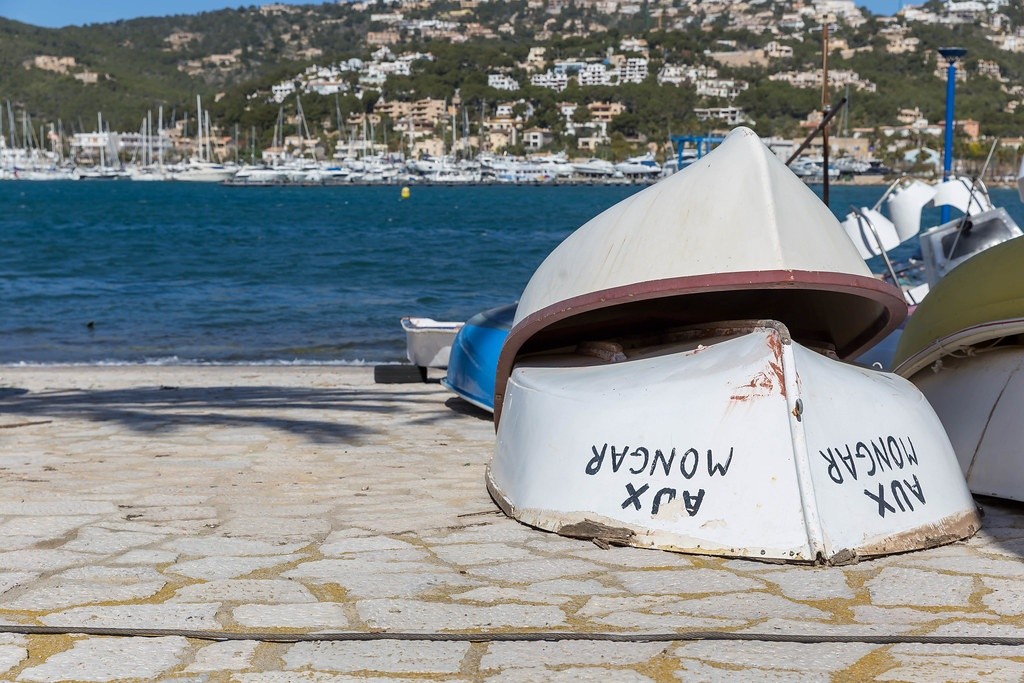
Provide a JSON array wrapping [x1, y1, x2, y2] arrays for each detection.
[[937, 47, 968, 225]]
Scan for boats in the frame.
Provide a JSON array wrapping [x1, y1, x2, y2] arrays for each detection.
[[837, 172, 1024, 373], [491, 123, 910, 440], [906, 334, 1024, 503], [439, 303, 519, 412], [401, 316, 466, 370], [483, 320, 987, 569]]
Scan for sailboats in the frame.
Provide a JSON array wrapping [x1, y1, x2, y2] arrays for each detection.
[[0, 93, 940, 186]]
